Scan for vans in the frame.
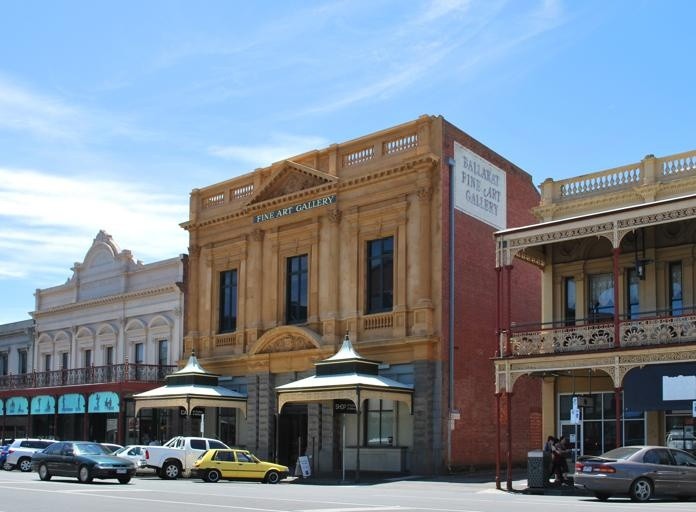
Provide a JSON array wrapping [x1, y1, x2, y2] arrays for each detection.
[[1, 438, 59, 472]]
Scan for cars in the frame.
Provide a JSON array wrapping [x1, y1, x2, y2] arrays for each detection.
[[572, 446, 696, 501], [30, 440, 157, 483], [191, 448, 290, 483], [667, 425, 696, 452]]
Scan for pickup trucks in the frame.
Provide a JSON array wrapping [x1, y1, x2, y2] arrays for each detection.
[[141, 436, 231, 479]]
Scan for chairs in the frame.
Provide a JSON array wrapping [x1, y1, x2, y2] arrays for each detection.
[[660, 457, 670, 465]]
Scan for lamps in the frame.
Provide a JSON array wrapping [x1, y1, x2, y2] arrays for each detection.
[[631, 225, 654, 282]]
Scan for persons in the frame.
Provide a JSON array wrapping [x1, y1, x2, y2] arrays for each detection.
[[543, 435, 576, 486], [143, 432, 160, 446]]
